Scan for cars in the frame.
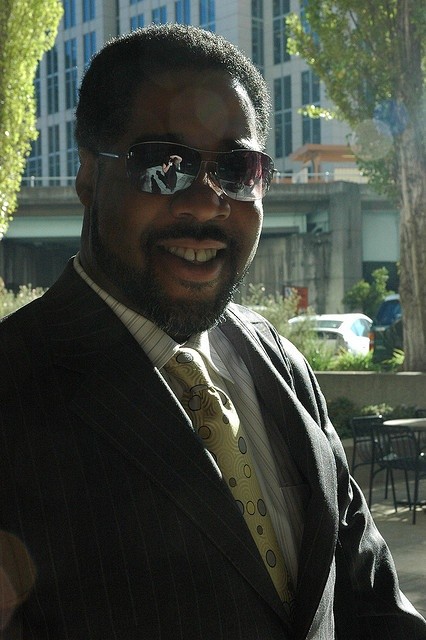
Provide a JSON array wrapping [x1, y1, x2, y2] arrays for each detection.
[[281, 312, 372, 364], [368, 295, 404, 364]]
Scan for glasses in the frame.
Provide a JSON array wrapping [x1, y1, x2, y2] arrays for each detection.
[[92, 142, 278, 202]]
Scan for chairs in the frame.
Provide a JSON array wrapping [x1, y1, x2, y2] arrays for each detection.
[[368, 423, 426, 525], [415, 409, 426, 448], [349, 415, 398, 514]]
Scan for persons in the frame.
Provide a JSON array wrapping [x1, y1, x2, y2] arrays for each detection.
[[0, 24, 426, 640], [162, 152, 182, 191]]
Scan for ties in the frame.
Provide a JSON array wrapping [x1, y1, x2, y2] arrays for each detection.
[[168, 346, 294, 608]]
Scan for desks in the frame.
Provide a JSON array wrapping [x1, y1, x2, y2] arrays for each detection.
[[383, 418, 426, 506]]
